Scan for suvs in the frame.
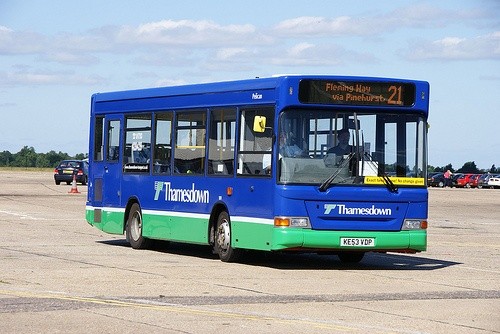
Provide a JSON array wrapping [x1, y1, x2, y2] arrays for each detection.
[[53, 160, 89, 186]]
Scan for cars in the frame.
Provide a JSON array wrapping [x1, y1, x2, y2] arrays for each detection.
[[384, 172, 500, 189]]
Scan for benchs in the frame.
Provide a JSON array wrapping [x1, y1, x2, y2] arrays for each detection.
[[111, 143, 172, 173]]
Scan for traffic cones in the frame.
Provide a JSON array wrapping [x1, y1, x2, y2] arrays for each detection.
[[68, 174, 81, 194]]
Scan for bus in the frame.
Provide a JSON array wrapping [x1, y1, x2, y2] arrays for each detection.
[[85, 75, 429, 263]]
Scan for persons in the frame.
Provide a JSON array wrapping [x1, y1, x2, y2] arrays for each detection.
[[326, 128, 361, 176], [263, 128, 308, 175]]
